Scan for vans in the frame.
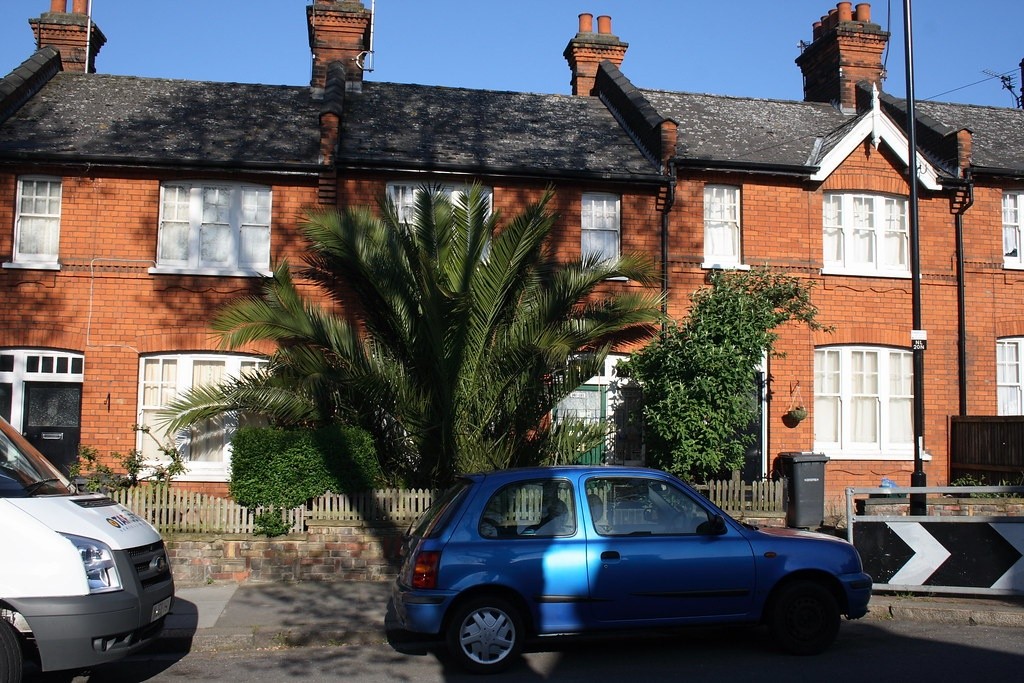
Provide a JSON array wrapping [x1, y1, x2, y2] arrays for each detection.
[[0, 414, 176, 683]]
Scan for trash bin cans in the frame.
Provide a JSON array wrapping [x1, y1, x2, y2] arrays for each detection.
[[774, 451, 830, 528]]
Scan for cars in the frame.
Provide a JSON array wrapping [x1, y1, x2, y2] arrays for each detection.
[[390, 466, 873, 676]]
